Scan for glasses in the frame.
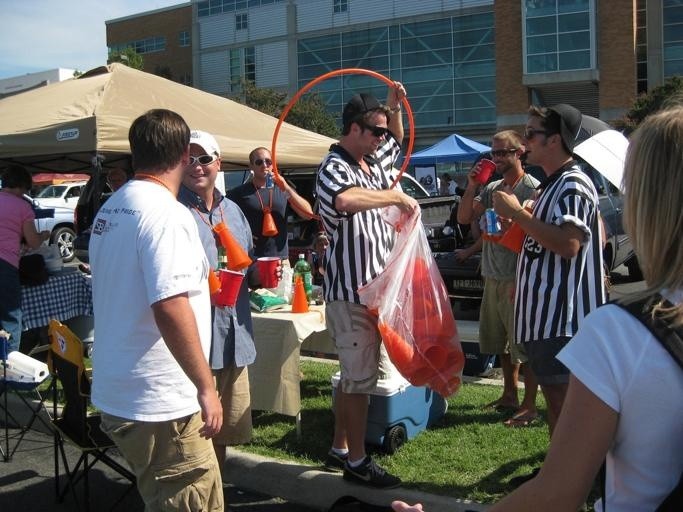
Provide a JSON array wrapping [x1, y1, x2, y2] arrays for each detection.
[[523, 129, 545, 138], [255, 159, 272, 166], [188, 156, 218, 167], [490, 149, 516, 155], [364, 123, 388, 137]]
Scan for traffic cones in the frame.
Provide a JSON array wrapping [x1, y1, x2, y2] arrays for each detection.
[[207, 266, 221, 296], [497, 206, 533, 254], [214, 223, 252, 271], [365, 250, 463, 399], [290, 277, 310, 314], [261, 206, 279, 237]]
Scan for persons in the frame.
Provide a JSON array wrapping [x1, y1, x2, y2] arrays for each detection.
[[176, 130, 282, 476], [88, 109, 224, 512], [316, 81, 418, 489], [391, 106, 683, 512], [492, 104, 606, 487], [225, 147, 313, 268], [440, 173, 458, 195], [455, 219, 482, 264], [311, 231, 329, 286], [0, 165, 50, 360], [457, 129, 543, 426]]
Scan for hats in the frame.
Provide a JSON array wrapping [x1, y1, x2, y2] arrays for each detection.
[[551, 103, 584, 154], [188, 130, 221, 157]]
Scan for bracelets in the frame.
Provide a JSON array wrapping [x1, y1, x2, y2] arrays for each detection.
[[389, 107, 400, 114], [512, 208, 524, 220]]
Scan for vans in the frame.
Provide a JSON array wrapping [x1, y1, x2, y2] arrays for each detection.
[[72, 163, 184, 261]]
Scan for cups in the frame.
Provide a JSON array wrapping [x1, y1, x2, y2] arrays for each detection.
[[214, 269, 245, 306], [256, 257, 279, 288], [473, 158, 496, 184], [313, 287, 325, 305]]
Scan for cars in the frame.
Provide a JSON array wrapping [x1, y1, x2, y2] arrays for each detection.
[[36, 181, 88, 208]]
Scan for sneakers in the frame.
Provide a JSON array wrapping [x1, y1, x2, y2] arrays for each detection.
[[325, 450, 352, 472], [341, 456, 403, 490]]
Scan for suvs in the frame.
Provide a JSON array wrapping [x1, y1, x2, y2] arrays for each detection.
[[250, 166, 433, 282], [0, 186, 80, 261]]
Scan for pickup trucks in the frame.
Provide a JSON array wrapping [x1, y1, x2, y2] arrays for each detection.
[[440, 155, 648, 309]]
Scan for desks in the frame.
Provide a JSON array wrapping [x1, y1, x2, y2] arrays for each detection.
[[248, 300, 338, 443], [17, 270, 93, 375]]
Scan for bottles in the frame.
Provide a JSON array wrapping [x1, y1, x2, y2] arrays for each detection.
[[292, 254, 311, 305]]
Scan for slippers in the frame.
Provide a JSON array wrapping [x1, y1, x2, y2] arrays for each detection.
[[503, 410, 538, 428], [482, 398, 521, 414]]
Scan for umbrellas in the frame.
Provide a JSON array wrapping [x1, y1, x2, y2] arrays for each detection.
[[573, 114, 630, 195]]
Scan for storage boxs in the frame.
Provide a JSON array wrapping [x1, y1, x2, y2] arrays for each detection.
[[329, 364, 446, 448], [452, 318, 497, 379]]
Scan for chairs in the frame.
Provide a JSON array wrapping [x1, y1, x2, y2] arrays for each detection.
[[0, 330, 65, 464], [44, 318, 138, 510]]
